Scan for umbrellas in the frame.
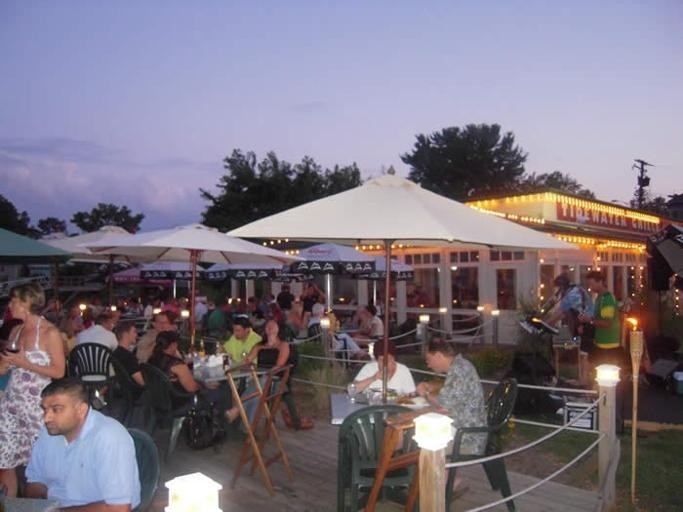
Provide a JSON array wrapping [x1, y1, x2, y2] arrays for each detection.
[[224, 168, 584, 512]]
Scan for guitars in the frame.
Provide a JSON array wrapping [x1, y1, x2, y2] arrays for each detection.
[[569, 308, 595, 353]]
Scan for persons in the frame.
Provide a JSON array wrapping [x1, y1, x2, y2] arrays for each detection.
[[0, 282, 66, 497], [410, 333, 491, 505], [21, 375, 144, 512], [0, 271, 623, 428], [345, 336, 418, 498]]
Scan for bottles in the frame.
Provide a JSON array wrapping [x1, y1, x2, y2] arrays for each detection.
[[197, 339, 205, 357], [240, 352, 248, 368], [222, 355, 230, 371], [214, 342, 220, 357]]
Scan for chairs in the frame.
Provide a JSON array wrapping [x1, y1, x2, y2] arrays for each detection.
[[112, 308, 397, 367], [438, 371, 523, 512], [65, 343, 301, 457], [335, 403, 425, 512], [121, 424, 161, 511]]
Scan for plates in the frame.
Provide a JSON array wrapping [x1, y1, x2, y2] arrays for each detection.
[[370, 386, 395, 392], [401, 397, 426, 406]]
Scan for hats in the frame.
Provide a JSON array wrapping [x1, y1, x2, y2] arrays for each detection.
[[374, 340, 396, 358]]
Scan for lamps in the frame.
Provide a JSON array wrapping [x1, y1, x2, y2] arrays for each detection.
[[414, 411, 450, 511], [161, 470, 228, 512], [592, 363, 624, 512]]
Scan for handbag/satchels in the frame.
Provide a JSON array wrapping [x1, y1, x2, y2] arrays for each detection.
[[281, 405, 313, 429], [192, 350, 224, 378], [185, 400, 225, 449]]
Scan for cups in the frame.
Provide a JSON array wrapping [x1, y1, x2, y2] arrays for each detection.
[[184, 359, 193, 374], [347, 383, 356, 403]]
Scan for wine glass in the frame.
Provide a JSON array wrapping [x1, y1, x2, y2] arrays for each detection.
[[5, 341, 19, 370]]
